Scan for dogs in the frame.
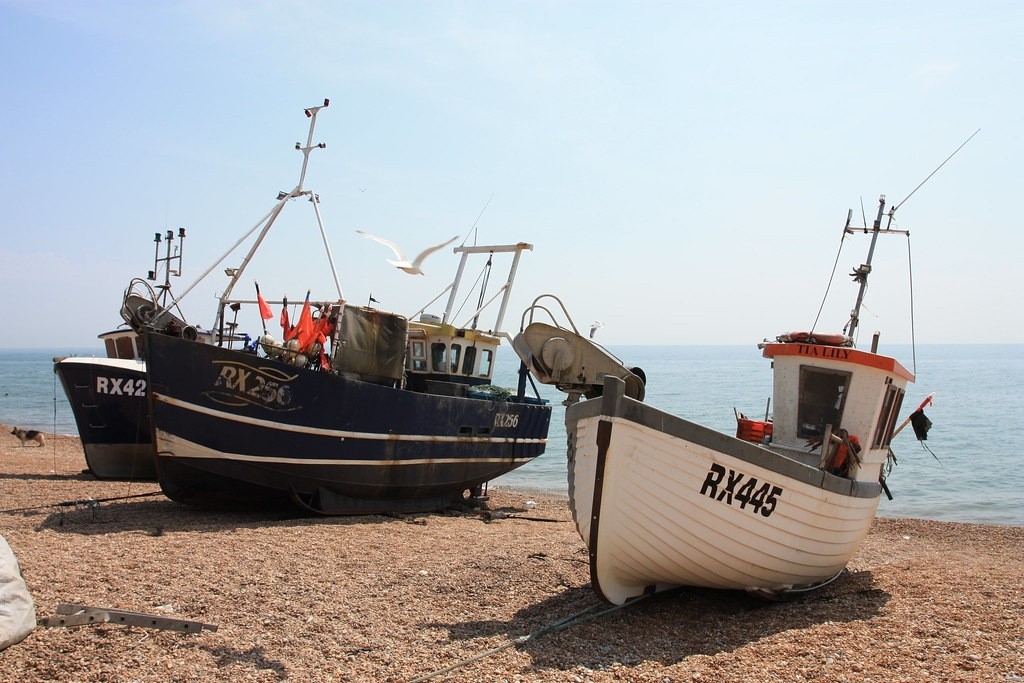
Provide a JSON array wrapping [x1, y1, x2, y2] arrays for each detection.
[[10, 427, 45, 447]]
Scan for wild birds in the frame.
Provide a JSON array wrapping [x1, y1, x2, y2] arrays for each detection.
[[355, 229, 460, 276]]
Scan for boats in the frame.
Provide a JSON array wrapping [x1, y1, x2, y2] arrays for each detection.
[[565, 129, 981, 607], [137, 98, 553, 516], [52, 229, 251, 482]]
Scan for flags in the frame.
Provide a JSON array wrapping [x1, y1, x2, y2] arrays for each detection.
[[257, 292, 333, 352]]
